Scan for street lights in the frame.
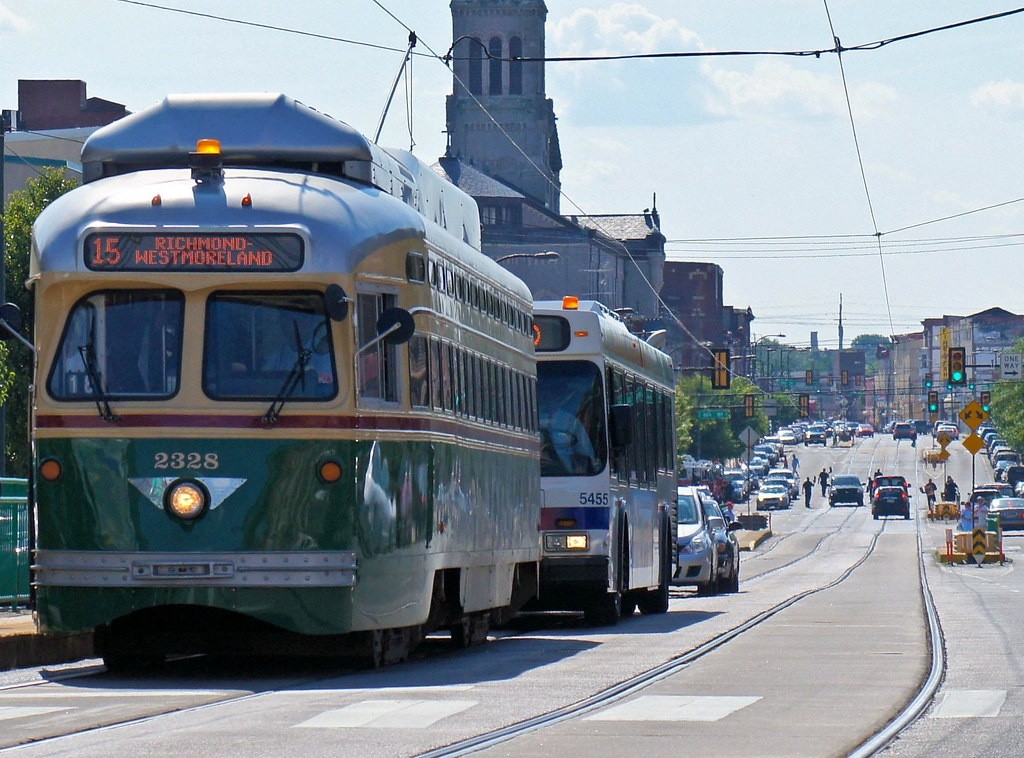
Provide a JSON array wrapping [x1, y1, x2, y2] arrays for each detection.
[[754, 334, 786, 385]]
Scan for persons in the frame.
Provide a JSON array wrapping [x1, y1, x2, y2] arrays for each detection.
[[538, 380, 594, 474], [974, 496, 990, 522], [725, 481, 734, 501], [868, 469, 882, 488], [723, 502, 734, 521], [818, 468, 829, 497], [911, 431, 917, 447], [925, 479, 937, 509], [960, 503, 972, 531], [792, 454, 800, 477], [262, 299, 332, 383], [802, 477, 814, 508], [945, 478, 958, 501], [781, 455, 788, 469]]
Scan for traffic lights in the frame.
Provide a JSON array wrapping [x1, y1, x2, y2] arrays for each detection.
[[925, 373, 932, 387], [927, 391, 939, 413], [948, 348, 966, 385], [981, 391, 990, 414]]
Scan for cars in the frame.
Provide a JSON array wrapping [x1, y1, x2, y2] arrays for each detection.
[[872, 476, 912, 494], [978, 422, 1021, 468], [675, 488, 717, 596], [988, 498, 1024, 531], [690, 485, 714, 499], [992, 460, 1017, 482], [803, 417, 959, 445], [826, 475, 866, 506], [721, 419, 808, 511], [872, 486, 912, 520], [701, 499, 743, 593], [961, 465, 1024, 509]]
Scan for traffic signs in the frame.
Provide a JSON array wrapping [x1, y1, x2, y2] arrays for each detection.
[[999, 353, 1021, 380]]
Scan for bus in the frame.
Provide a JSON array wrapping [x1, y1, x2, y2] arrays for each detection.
[[530, 298, 676, 628], [25, 92, 544, 673]]
